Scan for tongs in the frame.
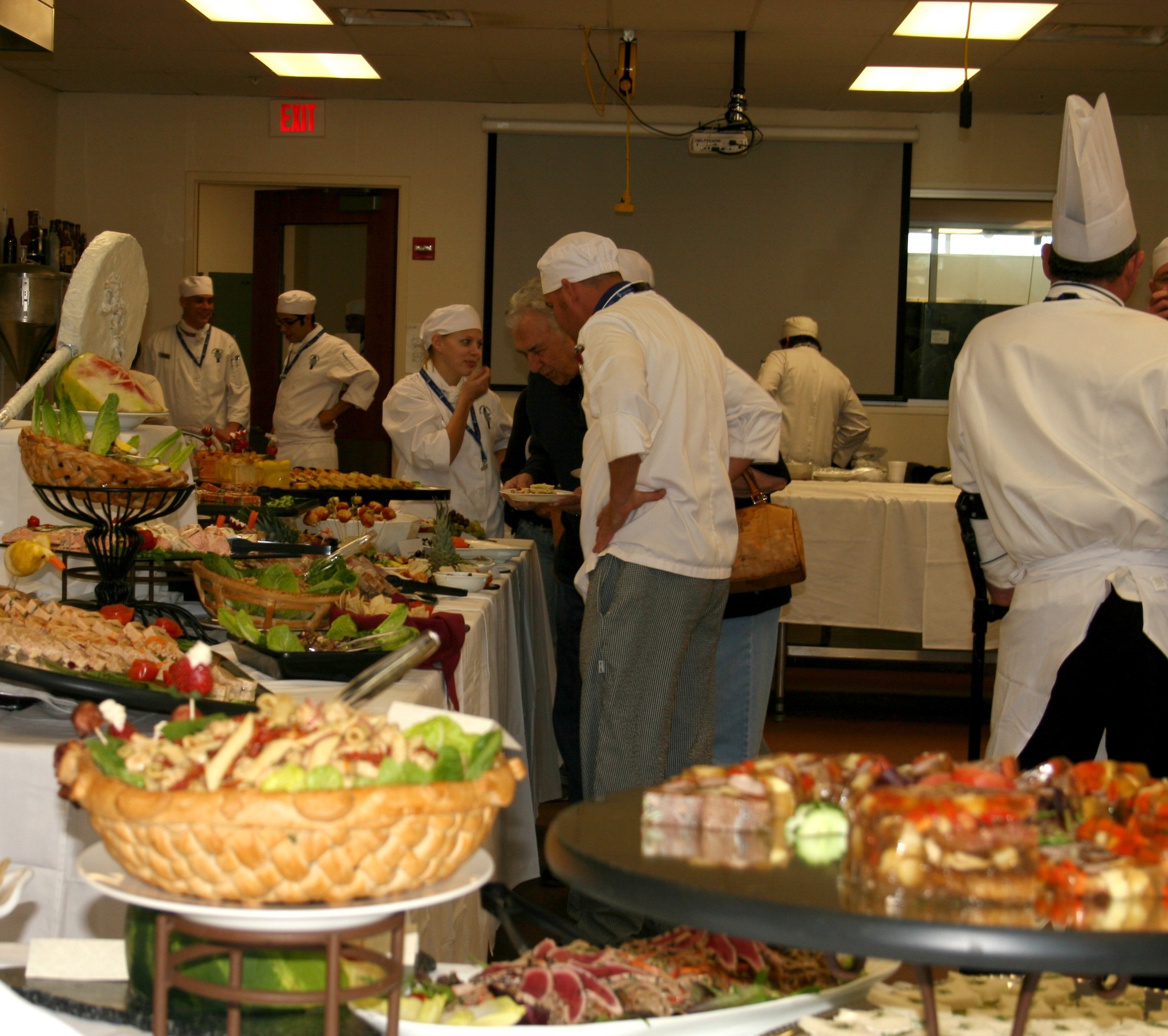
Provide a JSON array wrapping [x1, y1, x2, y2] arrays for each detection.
[[297, 530, 378, 582], [336, 628, 440, 714], [226, 537, 331, 557], [385, 574, 468, 597]]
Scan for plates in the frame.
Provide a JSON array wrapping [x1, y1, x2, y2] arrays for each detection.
[[30, 406, 170, 432], [346, 957, 903, 1035], [499, 488, 578, 503], [260, 467, 451, 501], [74, 840, 497, 933], [380, 567, 408, 572], [224, 628, 395, 681], [0, 628, 262, 716], [812, 471, 851, 481]]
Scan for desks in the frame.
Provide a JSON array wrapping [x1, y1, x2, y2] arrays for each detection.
[[1, 538, 564, 968], [771, 478, 1001, 719]]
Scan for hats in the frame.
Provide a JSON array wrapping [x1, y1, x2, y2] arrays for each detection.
[[1051, 92, 1137, 262], [537, 231, 619, 294], [781, 315, 818, 340], [419, 304, 482, 350], [616, 249, 655, 288], [1152, 237, 1168, 278], [179, 275, 214, 298], [277, 290, 316, 315]]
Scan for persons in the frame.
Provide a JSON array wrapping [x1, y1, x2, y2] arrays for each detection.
[[265, 291, 380, 472], [946, 93, 1168, 785], [136, 275, 251, 454], [501, 277, 586, 807], [381, 303, 513, 539], [758, 315, 872, 470], [538, 233, 793, 957], [1149, 236, 1168, 320]]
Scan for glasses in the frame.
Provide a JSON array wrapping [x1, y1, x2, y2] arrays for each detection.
[[1155, 274, 1168, 284], [273, 317, 304, 327]]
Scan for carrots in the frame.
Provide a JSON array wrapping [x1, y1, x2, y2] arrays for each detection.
[[48, 556, 66, 571], [216, 515, 226, 527], [247, 510, 258, 529]]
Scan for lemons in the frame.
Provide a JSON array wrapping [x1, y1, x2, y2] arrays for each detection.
[[3, 535, 51, 576]]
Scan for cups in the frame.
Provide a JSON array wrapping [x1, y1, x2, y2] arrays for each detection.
[[887, 461, 908, 483]]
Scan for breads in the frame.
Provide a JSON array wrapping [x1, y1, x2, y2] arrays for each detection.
[[290, 467, 416, 489]]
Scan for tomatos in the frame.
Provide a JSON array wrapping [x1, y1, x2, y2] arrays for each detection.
[[127, 530, 157, 550], [27, 515, 40, 528], [100, 603, 211, 695]]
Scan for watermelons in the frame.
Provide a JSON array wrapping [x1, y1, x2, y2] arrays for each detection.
[[53, 353, 166, 413]]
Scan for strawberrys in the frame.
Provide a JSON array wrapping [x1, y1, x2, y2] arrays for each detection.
[[302, 500, 397, 528]]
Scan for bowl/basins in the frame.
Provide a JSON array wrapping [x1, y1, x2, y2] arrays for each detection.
[[440, 561, 497, 574], [424, 539, 531, 564], [296, 512, 421, 555], [432, 571, 490, 592], [785, 463, 813, 480]]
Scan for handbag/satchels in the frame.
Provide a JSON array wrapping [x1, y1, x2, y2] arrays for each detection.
[[729, 468, 806, 592]]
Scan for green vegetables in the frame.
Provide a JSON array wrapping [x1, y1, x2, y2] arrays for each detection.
[[199, 552, 419, 653], [87, 710, 503, 789], [30, 385, 196, 473]]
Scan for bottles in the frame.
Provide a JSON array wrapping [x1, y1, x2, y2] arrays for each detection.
[[3, 218, 17, 263], [21, 208, 85, 274]]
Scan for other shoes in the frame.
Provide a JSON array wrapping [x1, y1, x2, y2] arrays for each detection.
[[551, 785, 569, 802]]
